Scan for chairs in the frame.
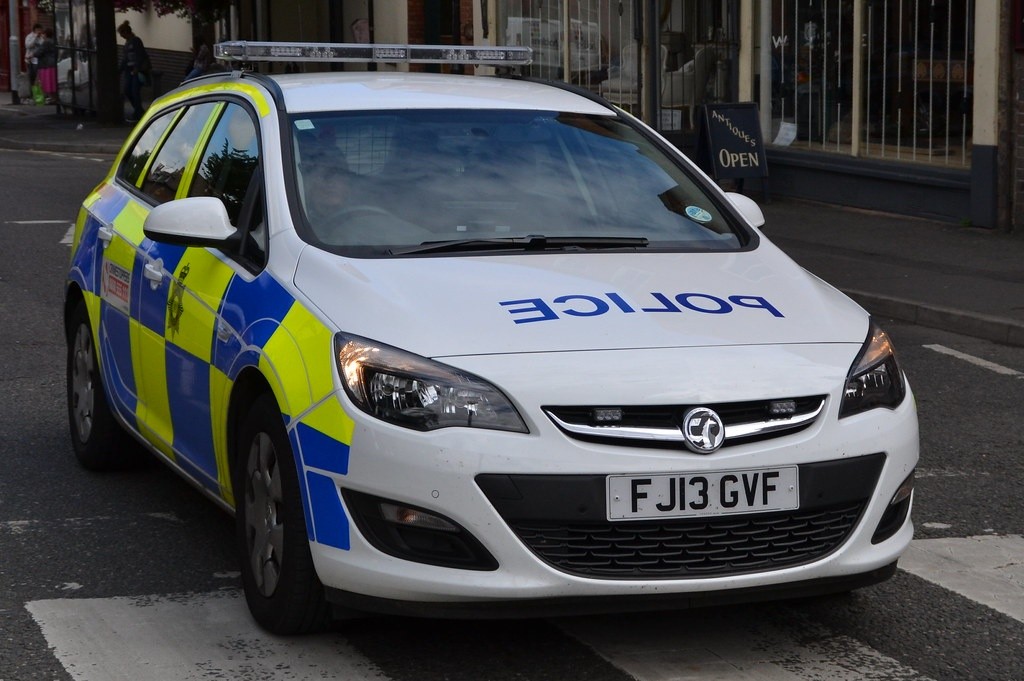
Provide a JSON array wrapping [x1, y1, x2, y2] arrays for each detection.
[[659, 45, 716, 129], [598, 42, 669, 104]]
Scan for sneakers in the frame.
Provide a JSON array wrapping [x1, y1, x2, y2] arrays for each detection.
[[126, 108, 146, 123]]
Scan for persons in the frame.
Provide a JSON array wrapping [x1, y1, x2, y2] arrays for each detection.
[[25, 24, 57, 104], [117, 21, 148, 123], [296, 143, 372, 221]]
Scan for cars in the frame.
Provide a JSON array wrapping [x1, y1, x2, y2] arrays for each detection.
[[63, 42, 919, 637]]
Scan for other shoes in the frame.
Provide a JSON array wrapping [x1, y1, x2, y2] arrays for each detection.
[[45, 98, 57, 104]]
